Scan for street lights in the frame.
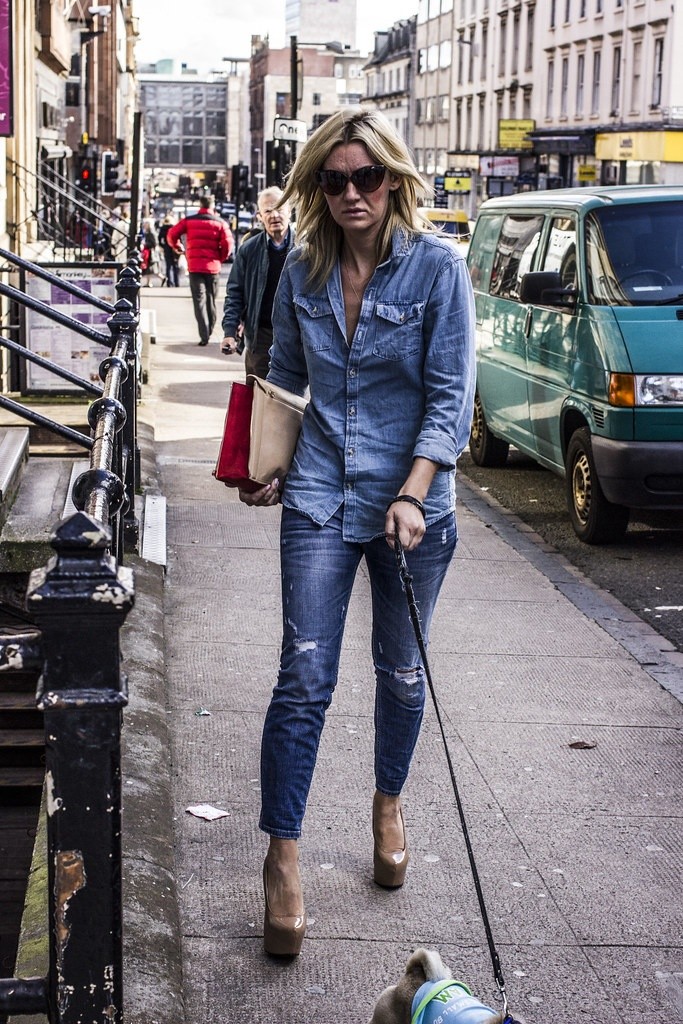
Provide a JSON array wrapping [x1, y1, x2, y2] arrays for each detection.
[[290, 36, 344, 168]]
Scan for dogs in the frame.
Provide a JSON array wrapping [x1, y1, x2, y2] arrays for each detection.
[[364, 946, 526, 1024]]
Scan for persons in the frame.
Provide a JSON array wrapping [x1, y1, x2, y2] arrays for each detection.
[[221, 187, 297, 386], [104, 212, 130, 269], [140, 196, 234, 346], [224, 108, 476, 955]]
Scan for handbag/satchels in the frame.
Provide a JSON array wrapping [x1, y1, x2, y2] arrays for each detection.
[[214, 375, 307, 496]]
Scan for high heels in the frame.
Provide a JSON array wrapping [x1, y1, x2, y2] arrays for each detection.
[[372, 806, 406, 886], [263, 857, 306, 954]]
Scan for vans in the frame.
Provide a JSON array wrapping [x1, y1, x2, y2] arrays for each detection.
[[464, 184, 683, 543], [417, 209, 470, 257]]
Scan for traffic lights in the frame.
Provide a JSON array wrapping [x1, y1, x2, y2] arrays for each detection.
[[102, 151, 119, 196], [79, 166, 93, 194]]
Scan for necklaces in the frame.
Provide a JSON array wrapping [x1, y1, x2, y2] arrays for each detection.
[[344, 255, 361, 304]]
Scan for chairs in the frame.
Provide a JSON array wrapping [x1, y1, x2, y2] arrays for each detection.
[[603, 224, 656, 288]]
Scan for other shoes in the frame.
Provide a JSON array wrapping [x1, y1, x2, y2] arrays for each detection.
[[199, 339, 208, 346]]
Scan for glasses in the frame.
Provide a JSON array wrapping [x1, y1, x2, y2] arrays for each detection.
[[315, 165, 386, 196]]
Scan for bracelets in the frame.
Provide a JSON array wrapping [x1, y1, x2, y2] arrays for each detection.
[[386, 495, 426, 520]]
